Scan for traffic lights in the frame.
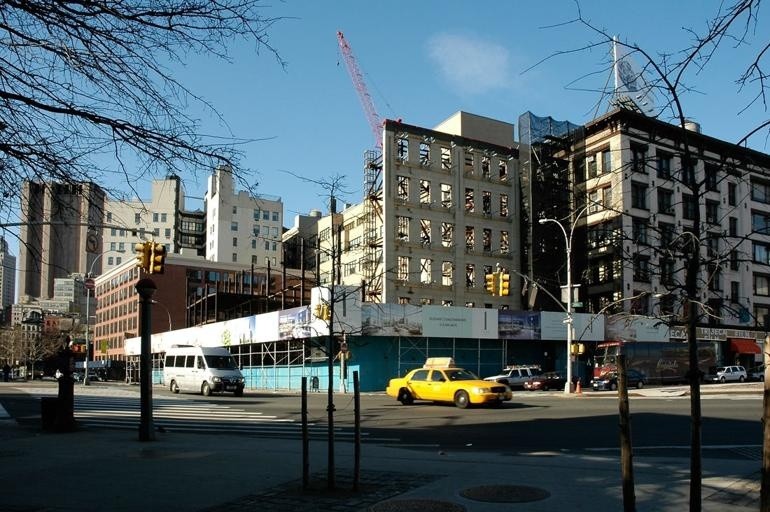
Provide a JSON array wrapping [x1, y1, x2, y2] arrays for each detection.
[[485, 271, 498, 296], [322, 304, 332, 320], [314, 304, 321, 317], [135, 241, 151, 270], [499, 271, 511, 297], [149, 240, 167, 275], [341, 342, 348, 352]]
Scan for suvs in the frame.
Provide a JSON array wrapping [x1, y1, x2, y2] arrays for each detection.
[[484, 364, 543, 391]]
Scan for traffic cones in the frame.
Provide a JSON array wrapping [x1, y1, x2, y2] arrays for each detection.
[[575, 380, 583, 394]]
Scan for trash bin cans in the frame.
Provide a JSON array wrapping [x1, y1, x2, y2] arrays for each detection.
[[40, 397, 58, 429]]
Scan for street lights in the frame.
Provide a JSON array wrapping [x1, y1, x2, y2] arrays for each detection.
[[84, 249, 127, 384], [312, 248, 337, 410], [538, 198, 605, 392], [151, 300, 172, 331]]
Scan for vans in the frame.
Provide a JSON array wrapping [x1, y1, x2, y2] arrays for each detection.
[[162, 346, 248, 397], [715, 365, 748, 384]]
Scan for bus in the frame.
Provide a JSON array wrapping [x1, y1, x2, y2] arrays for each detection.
[[593, 339, 720, 384]]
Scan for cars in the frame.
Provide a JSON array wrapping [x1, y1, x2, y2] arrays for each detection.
[[523, 371, 566, 391], [749, 368, 764, 381], [590, 369, 647, 391], [0, 364, 110, 381], [384, 354, 513, 409]]
